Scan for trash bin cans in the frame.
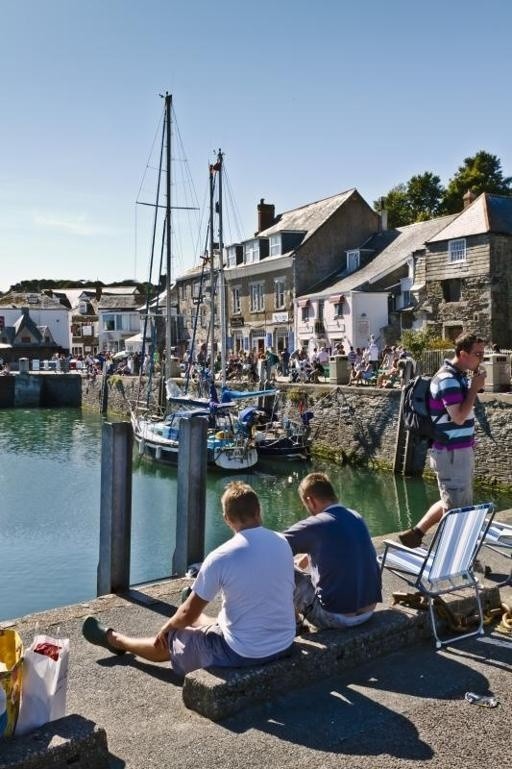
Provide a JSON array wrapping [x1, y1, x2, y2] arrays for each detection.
[[328, 355, 348, 385], [171, 357, 181, 378], [19, 357, 29, 373], [479, 352, 508, 393]]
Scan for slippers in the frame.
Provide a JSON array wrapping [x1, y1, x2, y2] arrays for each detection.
[[82, 617, 127, 657], [182, 586, 193, 606]]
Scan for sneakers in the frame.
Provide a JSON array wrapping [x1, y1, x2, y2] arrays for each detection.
[[399, 529, 424, 550], [461, 559, 493, 580]]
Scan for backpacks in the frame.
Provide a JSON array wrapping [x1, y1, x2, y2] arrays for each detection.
[[401, 369, 464, 439]]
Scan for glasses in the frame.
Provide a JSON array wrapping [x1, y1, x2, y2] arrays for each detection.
[[467, 351, 484, 357]]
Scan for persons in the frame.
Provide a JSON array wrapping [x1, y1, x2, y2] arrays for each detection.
[[282, 472, 381, 633], [0, 362, 9, 377], [52, 343, 410, 388], [81, 480, 297, 678], [400, 333, 486, 548]]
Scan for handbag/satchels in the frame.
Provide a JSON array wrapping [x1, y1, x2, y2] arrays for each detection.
[[0, 627, 25, 737], [14, 620, 73, 736]]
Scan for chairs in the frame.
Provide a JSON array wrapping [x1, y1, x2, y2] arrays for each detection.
[[469, 519, 512, 590], [376, 502, 497, 649]]
